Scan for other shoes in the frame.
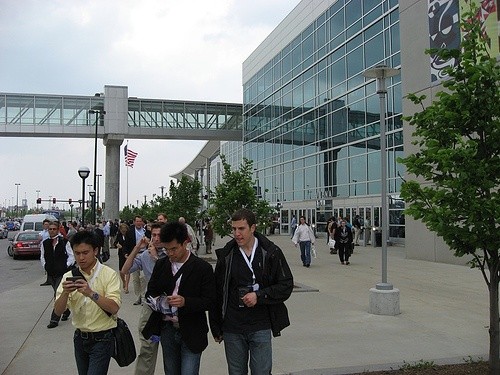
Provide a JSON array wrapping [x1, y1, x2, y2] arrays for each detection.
[[141, 298, 145, 306], [133, 298, 141, 305], [354, 244, 359, 246], [40, 280, 52, 286], [303, 263, 310, 267], [61, 311, 70, 321], [47, 322, 58, 328], [342, 262, 344, 264], [125, 287, 129, 294], [346, 262, 350, 265], [206, 250, 212, 254], [330, 250, 337, 254]]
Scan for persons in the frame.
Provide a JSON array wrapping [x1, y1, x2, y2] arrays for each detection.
[[135, 223, 168, 375], [291, 215, 315, 267], [334, 221, 354, 265], [326, 215, 374, 254], [54, 230, 124, 375], [141, 220, 214, 375], [0, 216, 22, 239], [208, 207, 294, 375], [39, 209, 217, 328], [290, 215, 297, 239]]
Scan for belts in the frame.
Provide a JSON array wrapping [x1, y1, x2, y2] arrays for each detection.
[[75, 329, 111, 340]]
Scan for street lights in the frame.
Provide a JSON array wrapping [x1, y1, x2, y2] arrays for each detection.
[[15, 183, 21, 218], [264, 188, 269, 236], [70, 205, 74, 221], [78, 109, 106, 229], [159, 186, 166, 205], [35, 190, 40, 213], [361, 63, 401, 316]]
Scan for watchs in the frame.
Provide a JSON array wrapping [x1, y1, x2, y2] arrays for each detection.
[[90, 292, 99, 302], [152, 256, 160, 262]]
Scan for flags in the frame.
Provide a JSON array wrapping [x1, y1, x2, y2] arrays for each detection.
[[124, 144, 139, 168]]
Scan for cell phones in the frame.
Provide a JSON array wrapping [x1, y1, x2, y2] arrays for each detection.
[[67, 276, 82, 283]]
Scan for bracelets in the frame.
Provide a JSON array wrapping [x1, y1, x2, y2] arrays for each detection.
[[135, 246, 140, 251]]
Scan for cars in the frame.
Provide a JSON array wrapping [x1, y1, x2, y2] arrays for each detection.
[[0, 216, 23, 238], [7, 231, 43, 259]]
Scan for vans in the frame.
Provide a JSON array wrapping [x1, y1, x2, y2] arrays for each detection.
[[20, 213, 60, 239]]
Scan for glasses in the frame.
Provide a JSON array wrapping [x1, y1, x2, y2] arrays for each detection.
[[49, 228, 58, 230]]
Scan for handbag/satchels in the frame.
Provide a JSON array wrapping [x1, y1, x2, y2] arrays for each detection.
[[311, 245, 316, 258], [109, 317, 137, 366], [328, 240, 336, 249]]
